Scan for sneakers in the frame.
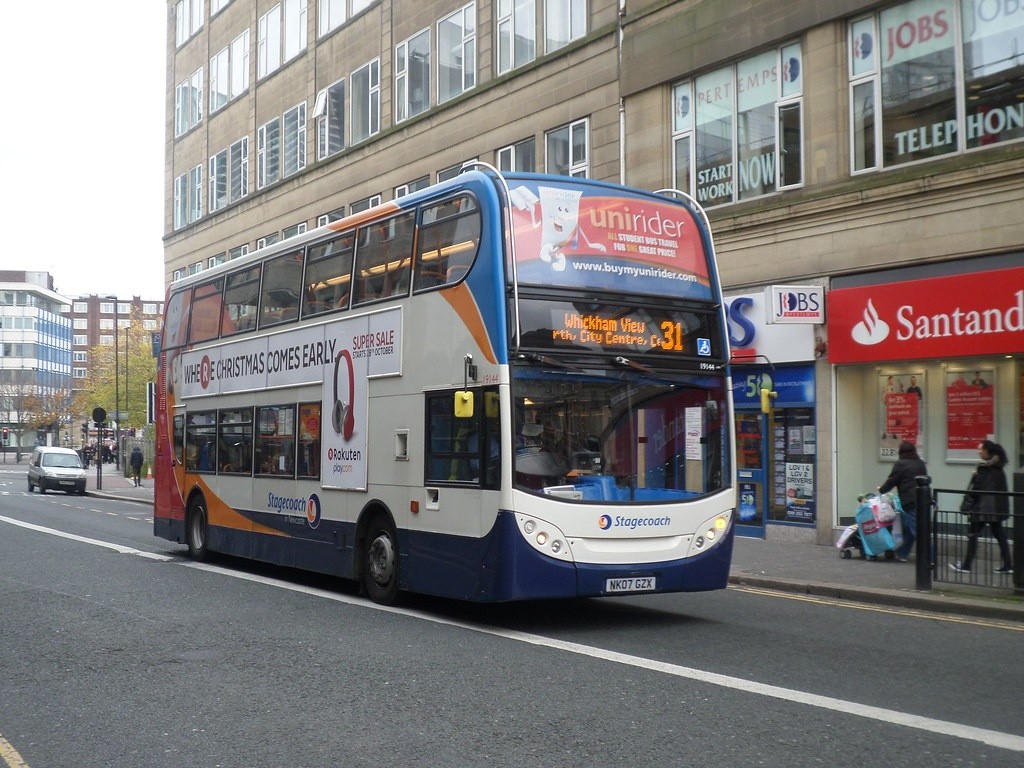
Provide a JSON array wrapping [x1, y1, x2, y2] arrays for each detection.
[[994, 566, 1014, 573], [949, 560, 971, 573], [932, 563, 934, 569], [894, 544, 911, 561]]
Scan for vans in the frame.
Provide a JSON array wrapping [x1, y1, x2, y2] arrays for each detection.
[[26, 443, 87, 494]]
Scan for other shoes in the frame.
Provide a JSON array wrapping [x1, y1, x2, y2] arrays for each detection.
[[139, 484, 144, 486], [135, 484, 137, 487]]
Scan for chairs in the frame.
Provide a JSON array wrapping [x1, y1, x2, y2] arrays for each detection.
[[261, 312, 279, 325], [339, 292, 381, 307], [236, 316, 255, 330], [223, 462, 241, 472], [279, 306, 299, 323], [447, 427, 468, 480], [447, 265, 471, 283], [304, 302, 332, 315], [418, 268, 446, 288]]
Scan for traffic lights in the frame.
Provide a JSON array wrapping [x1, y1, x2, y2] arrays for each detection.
[[2, 427, 9, 442]]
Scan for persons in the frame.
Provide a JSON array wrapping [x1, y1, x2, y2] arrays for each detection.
[[949, 440, 1014, 574], [881, 375, 922, 439], [876, 441, 935, 566], [84, 441, 118, 469], [951, 371, 988, 391], [130, 447, 144, 487]]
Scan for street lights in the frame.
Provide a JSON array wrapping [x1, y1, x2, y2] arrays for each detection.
[[63, 431, 74, 448]]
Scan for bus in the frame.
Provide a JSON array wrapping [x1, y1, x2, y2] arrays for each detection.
[[153, 157, 780, 605]]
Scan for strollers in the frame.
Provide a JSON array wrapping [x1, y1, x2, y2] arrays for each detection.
[[835, 487, 896, 560]]
